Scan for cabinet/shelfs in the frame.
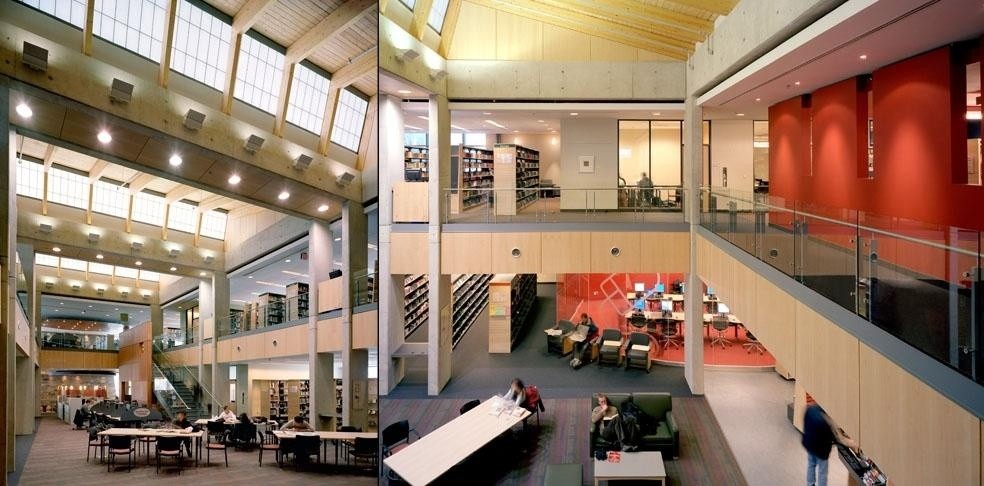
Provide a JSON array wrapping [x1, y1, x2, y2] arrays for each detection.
[[405, 144, 539, 215], [229, 277, 373, 334], [251, 379, 378, 431], [404, 274, 537, 353]]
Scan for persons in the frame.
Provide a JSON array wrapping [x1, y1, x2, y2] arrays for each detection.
[[81, 400, 95, 428], [279, 416, 315, 432], [637, 172, 654, 207], [173, 410, 194, 457], [592, 392, 640, 452], [218, 406, 236, 444], [570, 313, 599, 369], [502, 378, 528, 408], [669, 278, 684, 311], [166, 329, 176, 348], [801, 403, 857, 486], [228, 413, 250, 440]]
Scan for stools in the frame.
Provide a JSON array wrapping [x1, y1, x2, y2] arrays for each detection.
[[545, 464, 583, 486]]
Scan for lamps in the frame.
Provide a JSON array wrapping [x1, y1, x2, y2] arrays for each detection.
[[46, 283, 150, 299], [22, 41, 355, 187], [39, 224, 214, 264]]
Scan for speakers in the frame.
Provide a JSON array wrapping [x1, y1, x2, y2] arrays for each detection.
[[802, 93, 812, 107], [857, 73, 872, 91], [329, 269, 342, 279]]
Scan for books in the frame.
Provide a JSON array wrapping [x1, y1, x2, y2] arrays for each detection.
[[603, 413, 619, 420], [404, 152, 539, 209], [270, 380, 342, 416], [404, 274, 535, 347], [569, 325, 589, 342], [488, 398, 526, 417], [230, 282, 373, 334]]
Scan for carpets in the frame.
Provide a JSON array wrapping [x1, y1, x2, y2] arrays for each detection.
[[379, 396, 747, 486]]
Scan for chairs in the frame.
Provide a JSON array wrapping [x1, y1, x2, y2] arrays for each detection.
[[76, 409, 88, 430], [87, 417, 378, 475], [632, 392, 679, 460], [590, 393, 632, 457], [547, 293, 766, 373], [381, 386, 539, 486]]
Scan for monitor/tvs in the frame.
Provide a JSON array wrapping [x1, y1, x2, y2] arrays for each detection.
[[633, 298, 645, 309], [405, 167, 422, 181], [661, 299, 673, 311], [634, 282, 645, 291], [718, 302, 730, 313], [655, 282, 665, 292], [707, 285, 716, 294]]
